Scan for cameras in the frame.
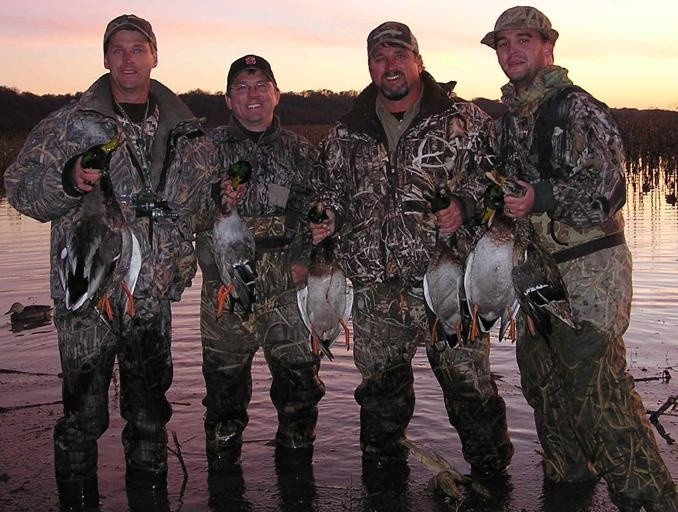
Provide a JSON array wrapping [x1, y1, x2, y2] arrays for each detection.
[[136, 199, 178, 219]]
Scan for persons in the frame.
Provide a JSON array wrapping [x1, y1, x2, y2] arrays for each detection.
[[188, 53, 331, 494], [4, 12, 201, 511], [319, 19, 520, 498], [478, 4, 678, 512]]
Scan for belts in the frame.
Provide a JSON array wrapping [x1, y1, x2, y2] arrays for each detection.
[[549, 228, 626, 264]]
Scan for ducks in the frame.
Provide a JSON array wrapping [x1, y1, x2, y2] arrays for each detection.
[[1, 300, 52, 322], [419, 190, 472, 350], [208, 158, 258, 322], [460, 167, 580, 352], [302, 200, 352, 363], [55, 133, 142, 327]]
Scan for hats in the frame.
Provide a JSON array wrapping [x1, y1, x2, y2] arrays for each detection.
[[366, 22, 419, 56], [480, 6, 559, 49], [226, 55, 277, 94], [104, 14, 157, 53]]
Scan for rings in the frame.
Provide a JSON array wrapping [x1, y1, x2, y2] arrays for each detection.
[[507, 208, 512, 213]]
